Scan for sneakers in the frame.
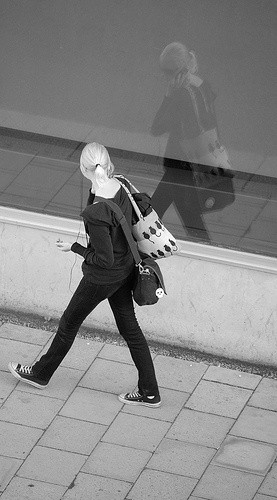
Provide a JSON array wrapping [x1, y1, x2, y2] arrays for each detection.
[[8, 361, 47, 390], [119, 390, 161, 407]]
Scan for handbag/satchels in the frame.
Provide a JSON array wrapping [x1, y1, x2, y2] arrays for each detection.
[[134, 259, 168, 306], [195, 176, 235, 213], [113, 174, 183, 259], [180, 128, 235, 190]]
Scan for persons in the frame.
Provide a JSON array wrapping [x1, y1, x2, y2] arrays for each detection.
[[149, 42, 218, 246], [8, 142, 162, 408]]
